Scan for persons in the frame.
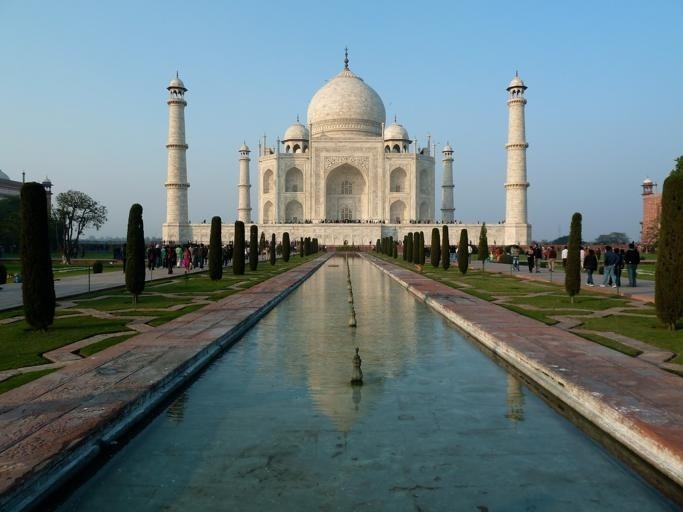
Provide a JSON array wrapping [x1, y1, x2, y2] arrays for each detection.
[[511, 244, 568, 274], [123, 243, 127, 272], [468, 244, 473, 264], [580, 243, 640, 288], [223, 245, 233, 266], [146, 243, 209, 275]]
